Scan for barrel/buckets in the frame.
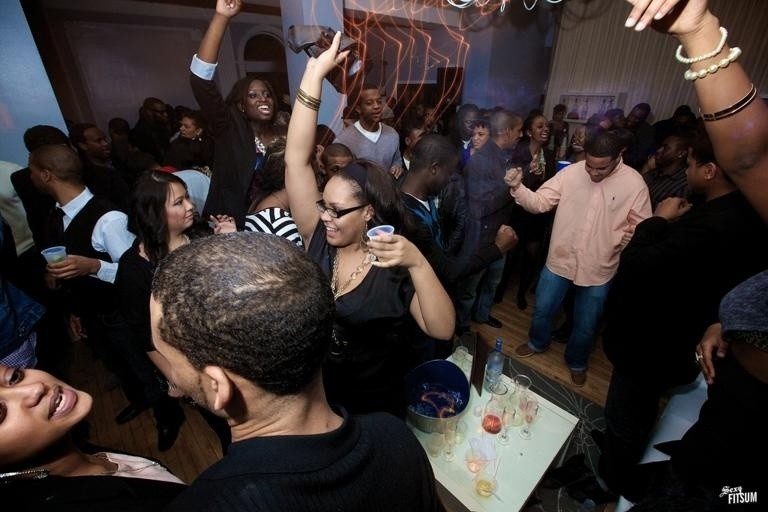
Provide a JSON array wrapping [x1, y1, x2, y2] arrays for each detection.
[[405, 359, 470, 434]]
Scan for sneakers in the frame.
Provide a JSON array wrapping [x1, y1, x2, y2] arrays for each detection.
[[516, 344, 534, 357], [484, 315, 505, 328], [569, 368, 586, 387], [566, 475, 617, 504], [591, 428, 607, 448]]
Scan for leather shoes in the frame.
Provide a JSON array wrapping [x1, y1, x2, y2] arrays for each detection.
[[115, 404, 143, 426], [155, 413, 186, 453]]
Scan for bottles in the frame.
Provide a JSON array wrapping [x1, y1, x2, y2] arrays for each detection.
[[482, 337, 507, 392], [482, 380, 508, 434], [510, 375, 533, 427]]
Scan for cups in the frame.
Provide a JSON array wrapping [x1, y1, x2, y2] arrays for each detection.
[[40, 246, 68, 264], [366, 222, 395, 263], [452, 334, 473, 370], [555, 160, 573, 171]]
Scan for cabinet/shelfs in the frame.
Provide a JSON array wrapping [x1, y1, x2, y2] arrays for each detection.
[[559, 91, 625, 126]]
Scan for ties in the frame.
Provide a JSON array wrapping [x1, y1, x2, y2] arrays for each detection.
[[54, 207, 65, 233]]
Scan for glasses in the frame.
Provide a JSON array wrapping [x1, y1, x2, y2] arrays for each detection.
[[314, 198, 370, 219], [150, 105, 166, 113]]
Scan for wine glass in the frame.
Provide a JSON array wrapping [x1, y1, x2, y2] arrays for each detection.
[[521, 401, 539, 439], [429, 416, 502, 502], [500, 406, 515, 444]]
